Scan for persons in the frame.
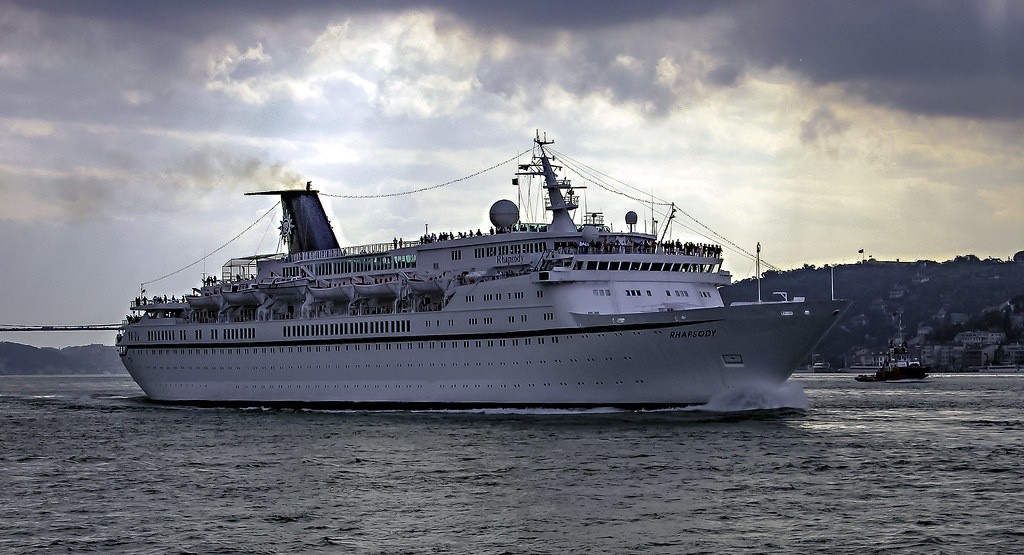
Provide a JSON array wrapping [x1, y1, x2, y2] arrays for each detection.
[[125, 225, 723, 323]]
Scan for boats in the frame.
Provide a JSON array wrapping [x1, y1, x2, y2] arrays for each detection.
[[352, 281, 401, 300], [856, 375, 877, 382], [309, 284, 356, 303], [222, 288, 268, 304], [877, 340, 929, 379], [408, 277, 455, 296], [185, 294, 222, 308], [116, 128, 857, 422], [257, 279, 309, 296]]
